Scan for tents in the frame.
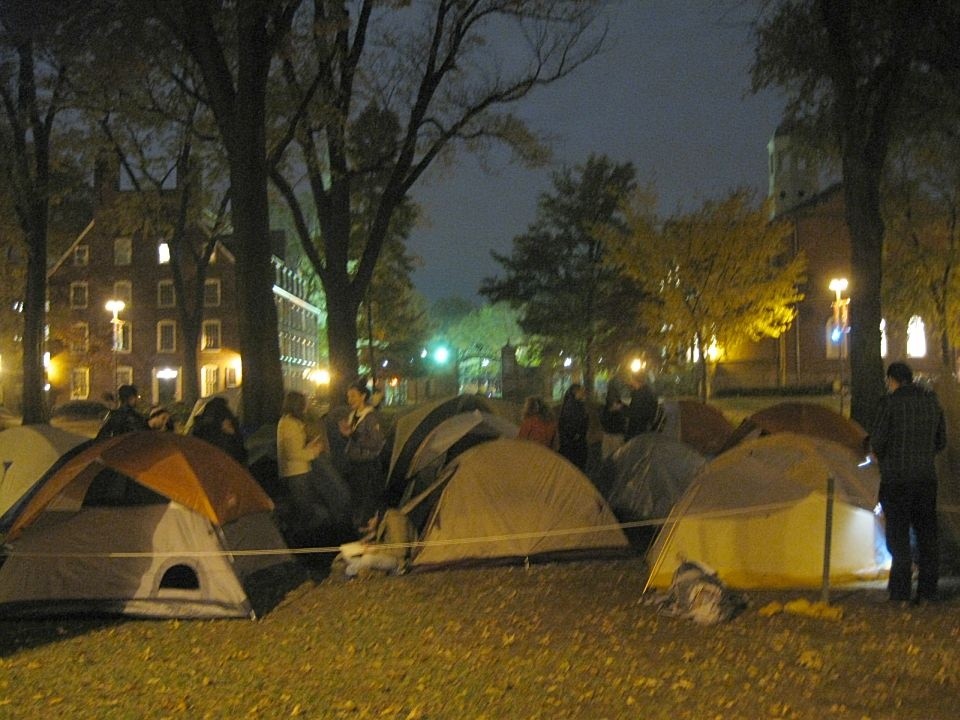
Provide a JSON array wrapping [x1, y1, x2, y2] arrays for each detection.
[[720, 403, 870, 457], [0, 411, 94, 521], [641, 430, 895, 603], [356, 419, 632, 576], [0, 429, 316, 623]]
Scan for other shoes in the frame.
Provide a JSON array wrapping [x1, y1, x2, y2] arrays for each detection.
[[915, 588, 954, 607], [887, 591, 912, 609]]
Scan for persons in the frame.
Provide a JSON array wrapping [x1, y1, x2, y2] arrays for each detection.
[[55, 355, 714, 519], [866, 361, 950, 608]]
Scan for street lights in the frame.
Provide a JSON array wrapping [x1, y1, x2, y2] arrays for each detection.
[[39, 269, 920, 418]]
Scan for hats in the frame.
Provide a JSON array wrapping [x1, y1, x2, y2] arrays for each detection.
[[118, 385, 139, 400]]
